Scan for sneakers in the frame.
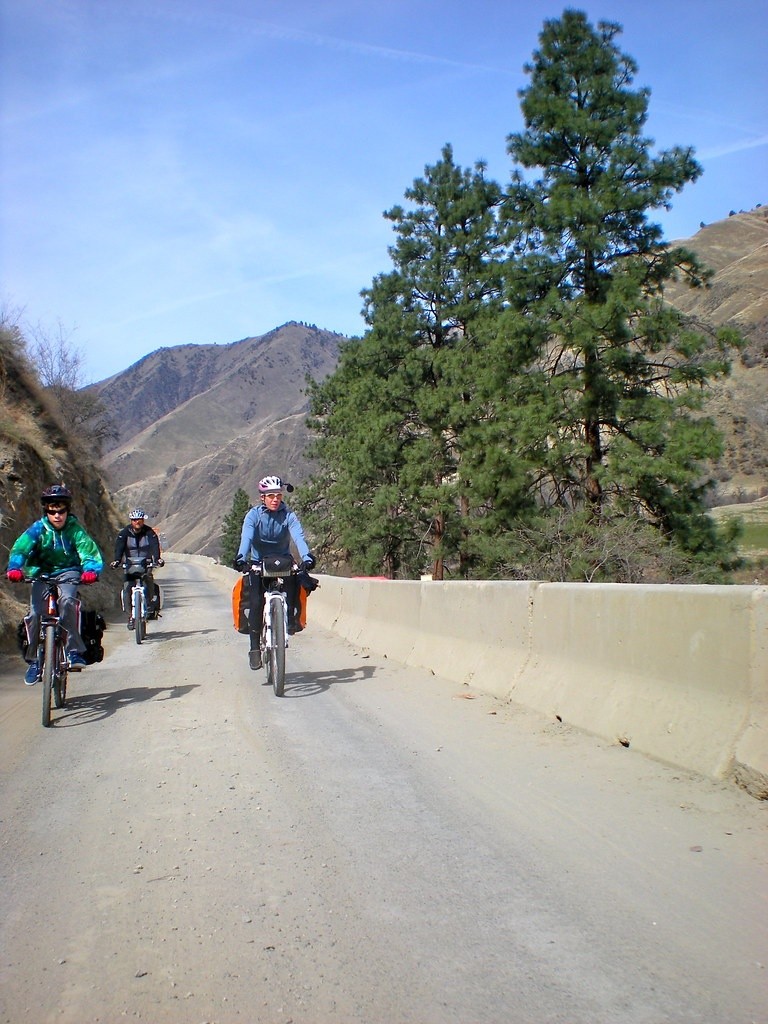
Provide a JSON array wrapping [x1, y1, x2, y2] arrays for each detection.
[[287, 614, 297, 635], [67, 652, 87, 668], [24, 662, 40, 686], [249, 649, 262, 670], [146, 607, 156, 618], [127, 617, 135, 630]]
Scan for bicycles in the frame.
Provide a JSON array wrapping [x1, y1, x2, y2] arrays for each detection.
[[234, 562, 312, 697], [3, 574, 100, 727], [111, 562, 163, 646]]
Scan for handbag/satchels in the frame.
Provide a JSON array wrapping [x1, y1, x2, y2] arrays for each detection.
[[232, 576, 252, 634], [152, 583, 165, 618], [79, 610, 106, 665], [290, 574, 320, 631]]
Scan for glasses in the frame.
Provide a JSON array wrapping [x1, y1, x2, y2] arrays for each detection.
[[48, 508, 66, 515], [266, 493, 282, 498]]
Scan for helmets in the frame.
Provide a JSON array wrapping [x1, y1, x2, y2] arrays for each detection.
[[129, 509, 145, 520], [40, 485, 72, 506], [259, 476, 285, 493]]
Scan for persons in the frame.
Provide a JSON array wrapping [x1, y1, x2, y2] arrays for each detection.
[[7, 486, 104, 686], [233, 476, 316, 671], [110, 510, 165, 631]]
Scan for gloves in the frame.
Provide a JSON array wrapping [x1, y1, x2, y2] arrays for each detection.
[[300, 562, 313, 572], [81, 572, 97, 585], [158, 558, 164, 567], [7, 569, 24, 582], [110, 561, 118, 570], [237, 560, 250, 574]]
